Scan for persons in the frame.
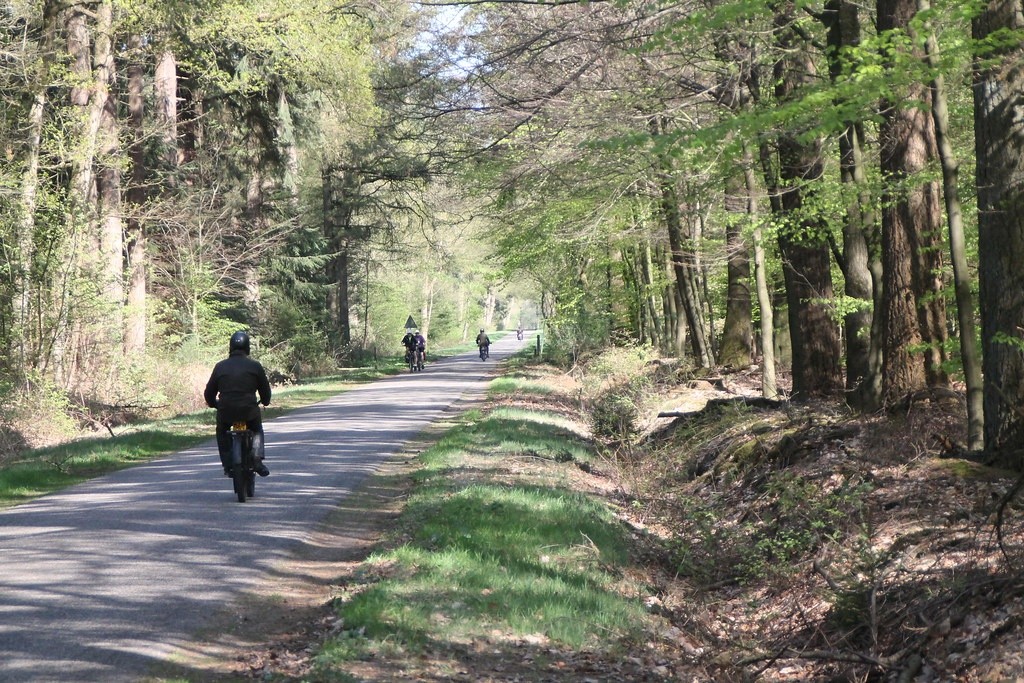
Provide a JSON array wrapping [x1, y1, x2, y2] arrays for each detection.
[[401, 329, 417, 366], [203, 332, 272, 478], [517, 327, 523, 335], [475, 328, 490, 358], [413, 330, 426, 369]]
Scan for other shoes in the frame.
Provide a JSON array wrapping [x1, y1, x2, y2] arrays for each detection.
[[406, 359, 409, 363], [480, 354, 482, 358], [414, 366, 417, 371], [487, 355, 489, 358], [251, 462, 269, 476], [422, 365, 424, 369]]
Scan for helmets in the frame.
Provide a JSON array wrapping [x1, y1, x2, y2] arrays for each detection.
[[230, 332, 250, 354], [480, 328, 484, 333], [414, 330, 420, 333], [407, 329, 412, 334]]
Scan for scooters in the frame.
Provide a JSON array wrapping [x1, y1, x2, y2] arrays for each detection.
[[403, 343, 425, 373], [476, 342, 492, 361], [206, 398, 263, 502], [517, 332, 524, 340]]
